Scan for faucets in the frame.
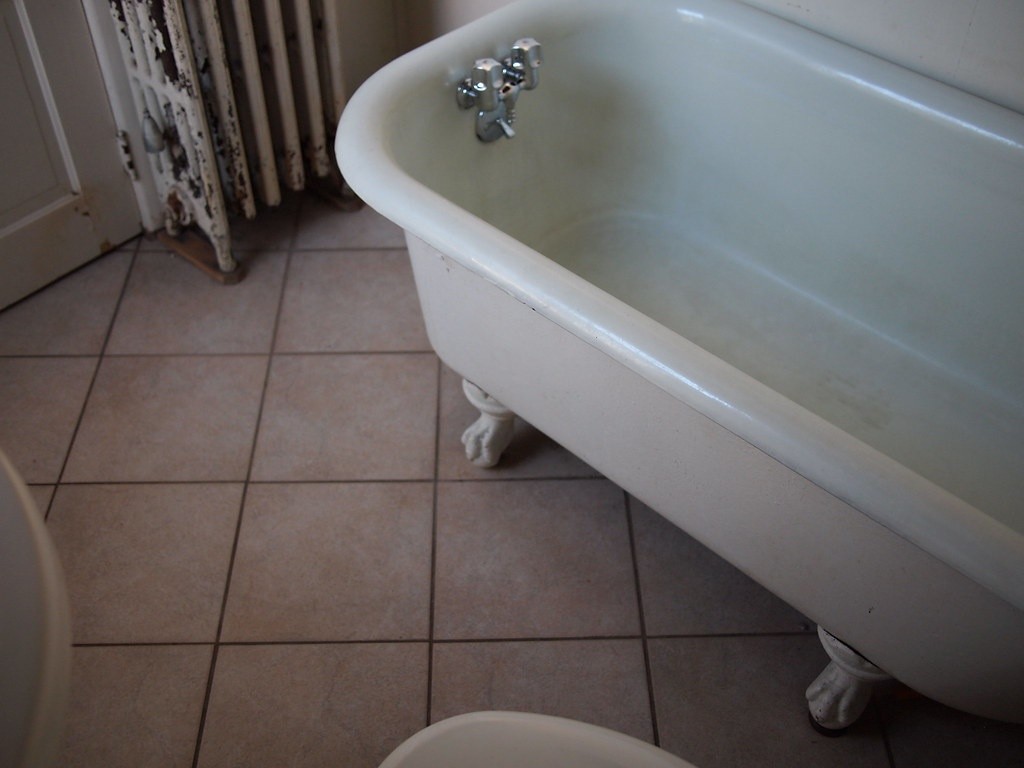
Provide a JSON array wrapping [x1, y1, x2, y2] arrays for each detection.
[[457, 36, 543, 143]]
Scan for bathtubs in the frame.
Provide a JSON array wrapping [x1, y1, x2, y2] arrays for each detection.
[[334, 1, 1024, 734]]
